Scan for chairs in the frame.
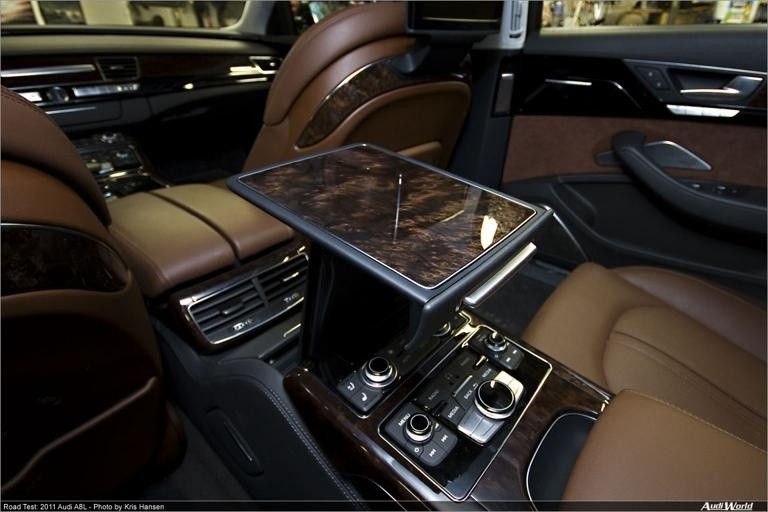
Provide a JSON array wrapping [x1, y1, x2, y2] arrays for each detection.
[[239, 1, 473, 177], [1, 86, 187, 498], [517, 258, 767, 448]]
